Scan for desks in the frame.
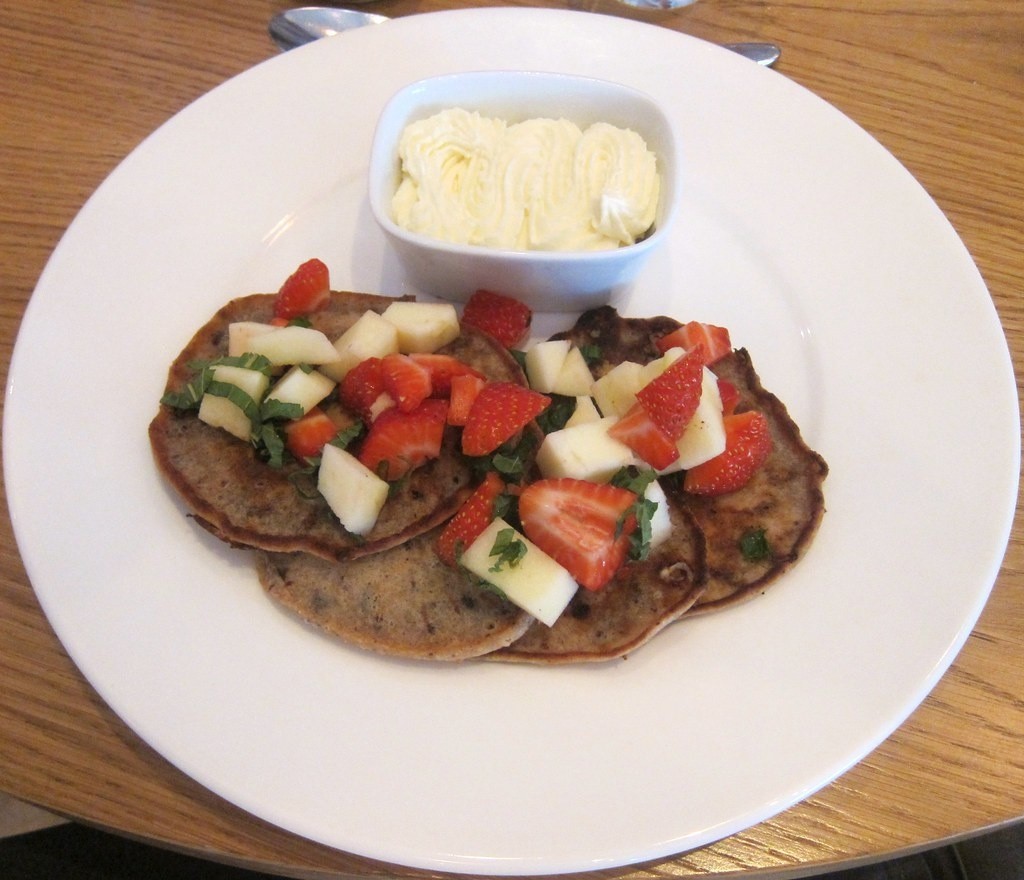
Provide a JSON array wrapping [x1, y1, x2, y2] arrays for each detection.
[[1, 0, 1023, 880]]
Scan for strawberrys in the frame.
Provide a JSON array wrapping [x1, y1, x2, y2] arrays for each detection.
[[270, 259, 773, 592]]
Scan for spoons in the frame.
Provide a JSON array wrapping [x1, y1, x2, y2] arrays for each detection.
[[267, 7, 780, 67]]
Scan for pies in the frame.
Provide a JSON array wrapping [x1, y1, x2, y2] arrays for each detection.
[[149, 290, 829, 664]]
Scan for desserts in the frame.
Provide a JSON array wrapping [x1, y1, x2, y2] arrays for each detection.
[[392, 108, 660, 252]]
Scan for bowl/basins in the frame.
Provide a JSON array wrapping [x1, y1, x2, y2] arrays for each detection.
[[368, 74, 681, 314]]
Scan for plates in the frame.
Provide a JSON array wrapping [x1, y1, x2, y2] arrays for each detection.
[[2, 7, 1019, 876]]
